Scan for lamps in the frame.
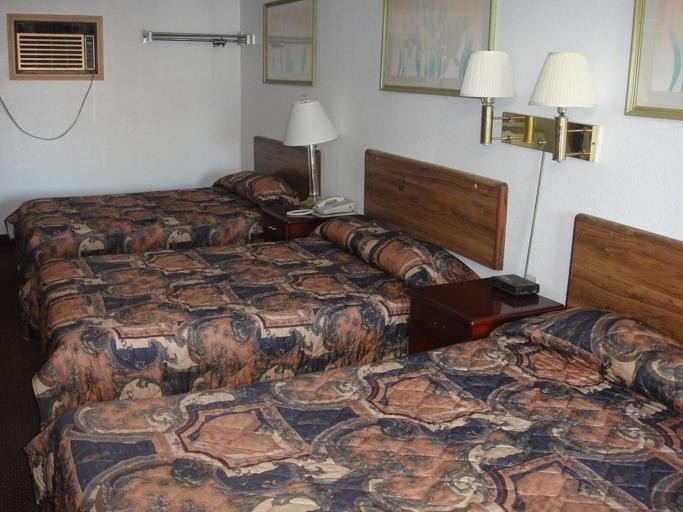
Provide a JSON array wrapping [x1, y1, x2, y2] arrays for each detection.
[[457, 49, 600, 164], [282, 97, 339, 209]]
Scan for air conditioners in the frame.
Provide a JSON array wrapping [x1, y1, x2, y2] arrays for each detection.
[[15, 31, 96, 73]]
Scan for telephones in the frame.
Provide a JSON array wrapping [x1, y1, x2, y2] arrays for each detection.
[[313, 195, 356, 216]]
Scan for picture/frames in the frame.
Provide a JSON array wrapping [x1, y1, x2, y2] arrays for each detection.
[[622, 0, 682, 120], [259, 0, 495, 102]]
